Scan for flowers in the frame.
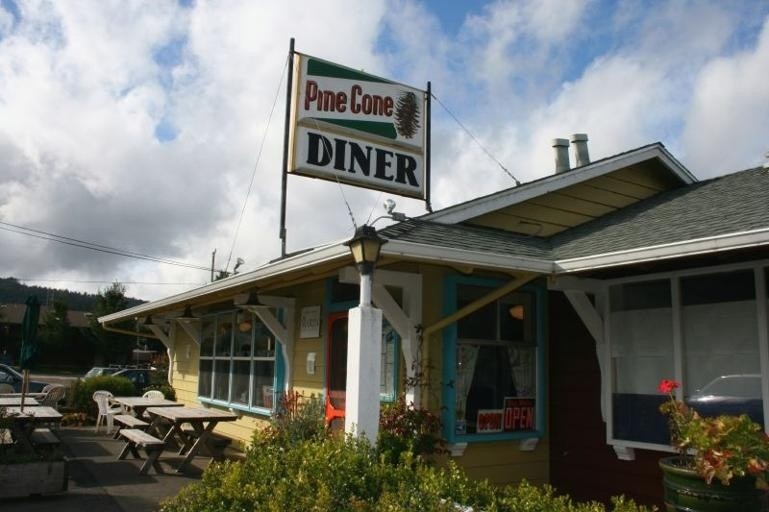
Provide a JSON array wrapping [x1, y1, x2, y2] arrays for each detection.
[[656, 379, 769, 485]]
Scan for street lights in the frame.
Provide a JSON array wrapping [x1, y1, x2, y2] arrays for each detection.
[[341, 224, 388, 448]]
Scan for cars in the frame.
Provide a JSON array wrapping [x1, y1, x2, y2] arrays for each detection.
[[687, 374, 763, 422], [0, 364, 50, 393], [83, 364, 160, 386]]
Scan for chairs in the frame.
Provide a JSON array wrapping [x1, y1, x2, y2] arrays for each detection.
[[0, 383, 15, 393], [142, 390, 165, 399], [93, 390, 128, 435], [35, 383, 66, 432]]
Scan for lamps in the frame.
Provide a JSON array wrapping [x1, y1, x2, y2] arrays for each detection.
[[239, 290, 270, 310], [179, 306, 200, 323], [140, 315, 155, 327]]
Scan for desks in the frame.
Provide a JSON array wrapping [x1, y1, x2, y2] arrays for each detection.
[[114, 397, 184, 431], [146, 407, 238, 472], [0, 392, 62, 461]]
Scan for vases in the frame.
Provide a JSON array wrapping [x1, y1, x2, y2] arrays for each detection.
[[657, 456, 760, 511]]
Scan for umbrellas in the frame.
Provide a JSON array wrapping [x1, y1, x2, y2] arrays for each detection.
[[16, 292, 41, 410]]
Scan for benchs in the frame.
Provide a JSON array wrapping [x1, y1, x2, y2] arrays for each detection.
[[179, 427, 232, 464], [117, 429, 168, 475]]
